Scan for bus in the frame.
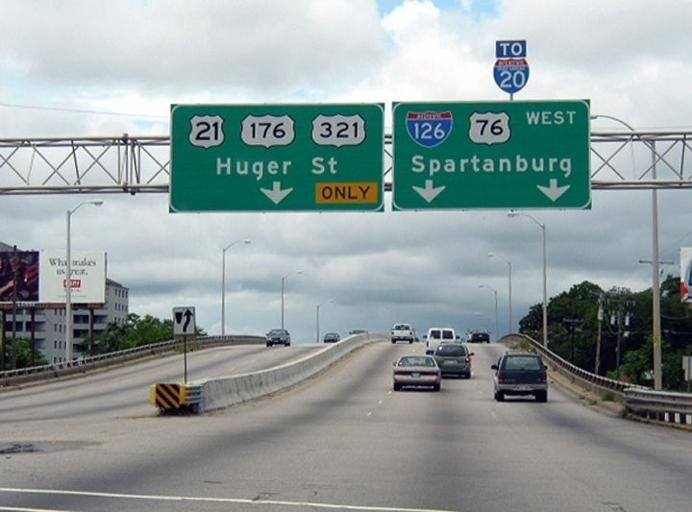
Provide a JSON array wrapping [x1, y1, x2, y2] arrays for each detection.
[[349, 329, 368, 334]]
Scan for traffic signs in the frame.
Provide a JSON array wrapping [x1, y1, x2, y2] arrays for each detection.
[[168, 104, 385, 211], [391, 101, 589, 209]]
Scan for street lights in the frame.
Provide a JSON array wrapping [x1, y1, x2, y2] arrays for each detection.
[[315, 298, 335, 341], [479, 284, 499, 338], [506, 210, 551, 350], [280, 271, 304, 329], [590, 113, 663, 385], [63, 198, 104, 361], [220, 238, 253, 334], [486, 251, 511, 327]]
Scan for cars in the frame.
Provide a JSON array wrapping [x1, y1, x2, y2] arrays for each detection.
[[265, 327, 291, 347], [386, 323, 547, 402], [325, 333, 340, 342]]
